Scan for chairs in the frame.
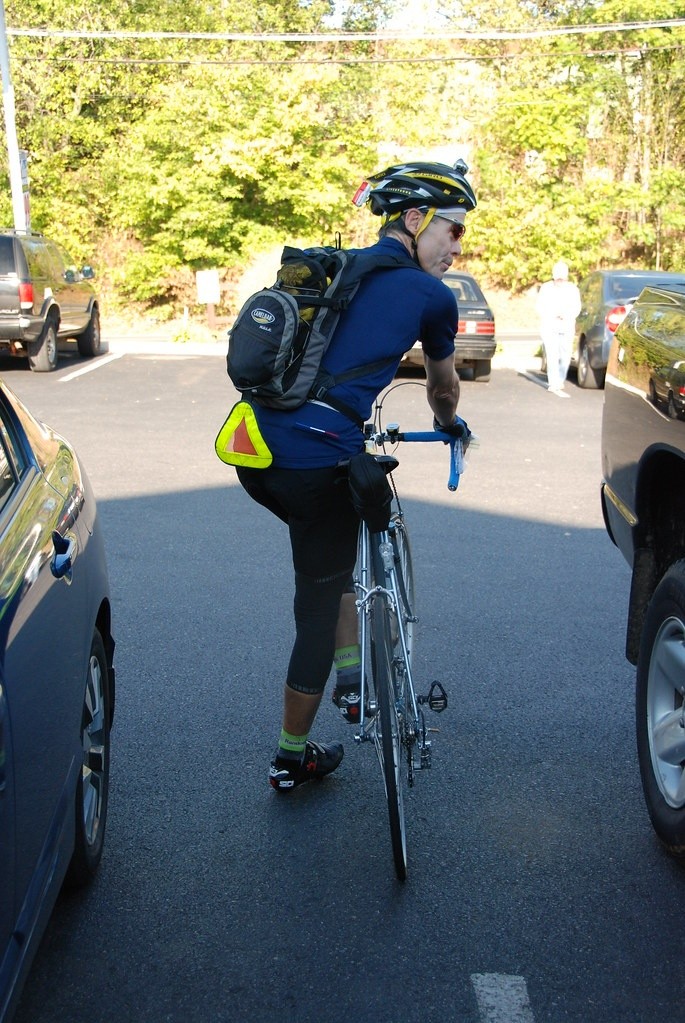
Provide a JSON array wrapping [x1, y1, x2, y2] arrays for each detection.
[[450, 288, 462, 300]]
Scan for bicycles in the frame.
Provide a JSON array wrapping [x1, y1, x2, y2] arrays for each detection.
[[353, 382, 464, 881]]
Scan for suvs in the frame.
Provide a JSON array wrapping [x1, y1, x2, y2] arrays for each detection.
[[0, 228, 101, 371]]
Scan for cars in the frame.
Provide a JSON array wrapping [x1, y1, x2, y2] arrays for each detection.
[[541, 270, 685, 388], [1, 376, 117, 1022], [398, 270, 496, 383]]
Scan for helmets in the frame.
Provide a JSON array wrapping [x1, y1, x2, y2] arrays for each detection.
[[353, 159, 477, 228]]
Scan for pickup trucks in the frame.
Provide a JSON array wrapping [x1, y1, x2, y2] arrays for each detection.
[[601, 284, 685, 858]]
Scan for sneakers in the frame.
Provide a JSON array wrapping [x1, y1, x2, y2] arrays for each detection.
[[332, 671, 369, 722], [269, 740, 344, 793]]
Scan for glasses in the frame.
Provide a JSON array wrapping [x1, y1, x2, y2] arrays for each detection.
[[404, 209, 466, 241]]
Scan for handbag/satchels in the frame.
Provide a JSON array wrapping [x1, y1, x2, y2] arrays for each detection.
[[347, 452, 393, 512]]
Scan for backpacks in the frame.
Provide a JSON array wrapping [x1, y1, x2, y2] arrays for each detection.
[[227, 245, 421, 411]]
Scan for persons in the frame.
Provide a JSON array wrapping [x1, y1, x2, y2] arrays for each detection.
[[214, 162, 476, 791], [537, 262, 581, 391]]
